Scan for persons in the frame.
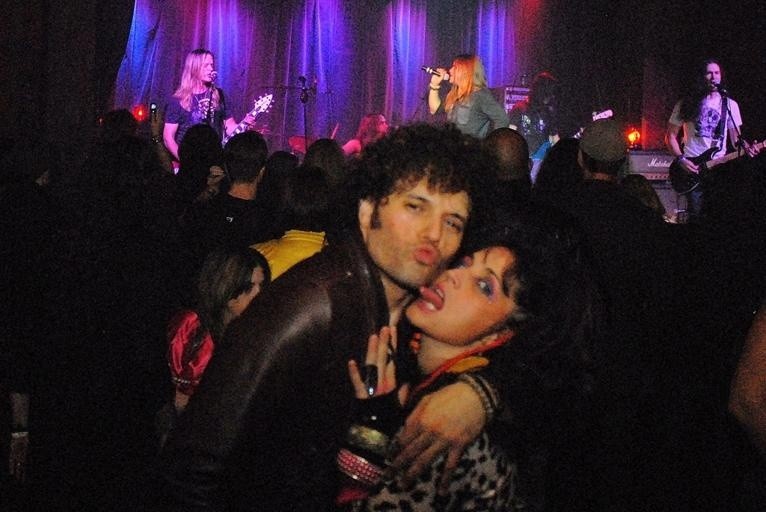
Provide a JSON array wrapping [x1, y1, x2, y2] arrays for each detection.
[[427, 53, 511, 140], [2, 102, 766, 511], [161, 48, 256, 168], [507, 73, 564, 161], [665, 58, 761, 221]]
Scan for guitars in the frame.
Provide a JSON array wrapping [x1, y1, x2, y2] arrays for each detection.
[[171, 94, 274, 174], [529, 109, 613, 184], [669, 139, 766, 195]]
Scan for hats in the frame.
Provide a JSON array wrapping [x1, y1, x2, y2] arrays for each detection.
[[579, 119, 627, 162], [484, 127, 529, 179]]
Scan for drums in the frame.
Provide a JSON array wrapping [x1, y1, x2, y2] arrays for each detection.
[[288, 135, 315, 168]]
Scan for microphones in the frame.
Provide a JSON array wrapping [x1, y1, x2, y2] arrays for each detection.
[[312, 78, 317, 104], [421, 66, 449, 80], [211, 71, 218, 91], [710, 80, 726, 95]]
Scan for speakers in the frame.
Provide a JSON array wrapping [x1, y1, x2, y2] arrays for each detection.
[[627, 150, 690, 227]]
